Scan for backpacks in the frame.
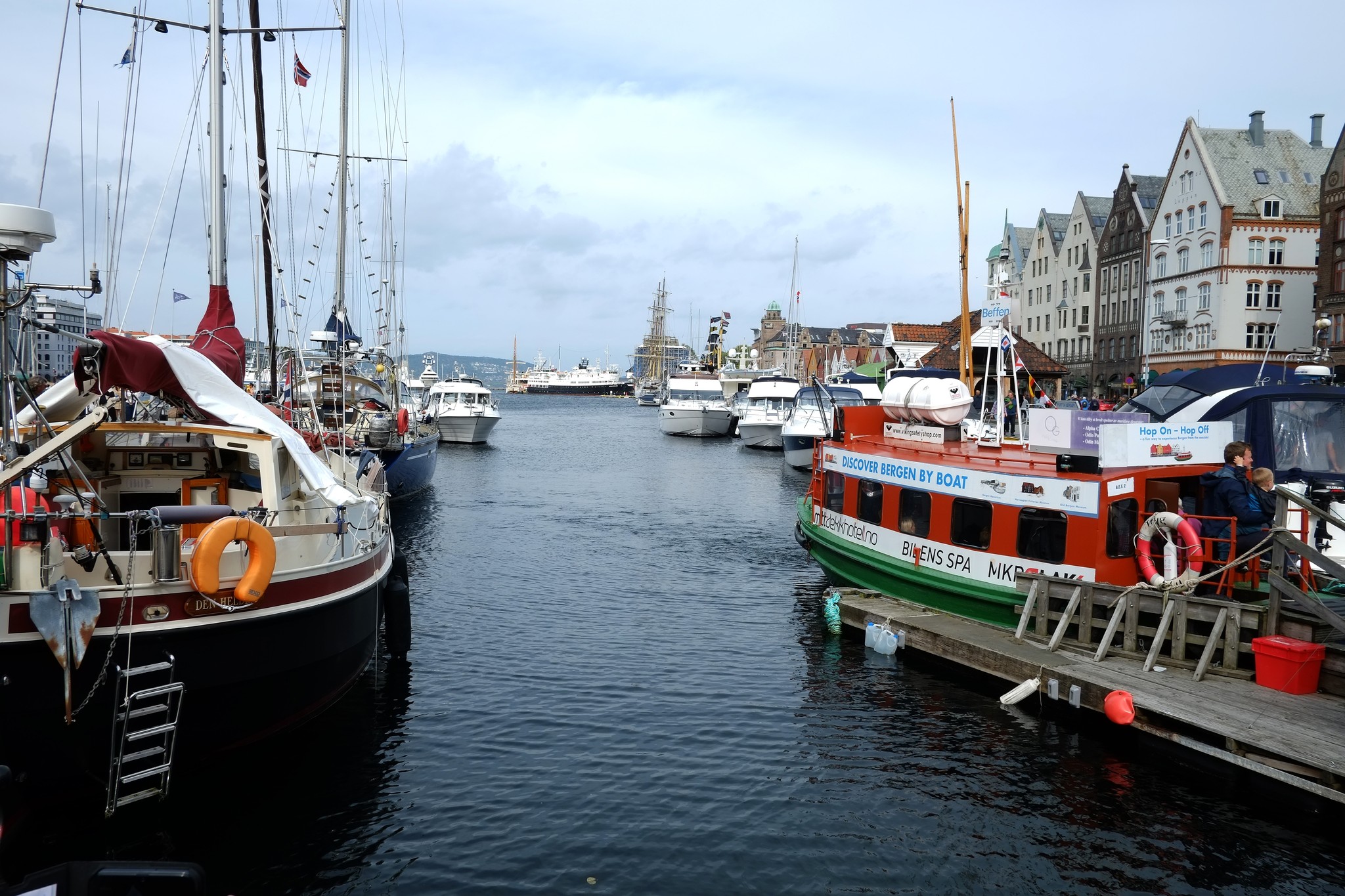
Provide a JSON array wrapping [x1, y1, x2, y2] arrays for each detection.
[[1082, 401, 1087, 408], [1092, 401, 1097, 407]]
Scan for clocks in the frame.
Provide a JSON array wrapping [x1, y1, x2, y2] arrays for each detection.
[[128, 452, 144, 466]]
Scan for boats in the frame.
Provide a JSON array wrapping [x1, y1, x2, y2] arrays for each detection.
[[425, 362, 501, 446], [637, 384, 660, 406], [504, 345, 631, 395], [628, 378, 635, 384], [659, 237, 1345, 665], [556, 372, 567, 376]]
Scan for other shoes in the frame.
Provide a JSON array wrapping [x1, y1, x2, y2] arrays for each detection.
[[1238, 565, 1249, 574], [1011, 433, 1015, 436], [1213, 559, 1224, 569], [1281, 592, 1296, 602], [1006, 430, 1009, 432]]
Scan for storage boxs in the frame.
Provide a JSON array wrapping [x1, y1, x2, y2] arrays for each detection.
[[1251, 635, 1325, 695]]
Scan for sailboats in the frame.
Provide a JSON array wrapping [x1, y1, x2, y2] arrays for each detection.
[[2, 1, 439, 893], [626, 271, 678, 398], [504, 334, 527, 394]]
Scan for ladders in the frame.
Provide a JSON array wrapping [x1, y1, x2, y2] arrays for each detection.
[[105, 653, 184, 815]]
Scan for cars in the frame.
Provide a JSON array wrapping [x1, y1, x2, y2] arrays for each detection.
[[1088, 399, 1116, 411], [1112, 401, 1121, 411]]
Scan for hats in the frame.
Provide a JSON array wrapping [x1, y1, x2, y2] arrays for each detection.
[[1083, 397, 1086, 399], [1122, 395, 1128, 397]]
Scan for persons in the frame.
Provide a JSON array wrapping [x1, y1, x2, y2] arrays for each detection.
[[1209, 456, 1277, 573], [616, 391, 618, 396], [624, 391, 634, 397], [1199, 441, 1290, 581], [249, 383, 254, 396], [1111, 395, 1128, 412], [1019, 393, 1055, 419], [1066, 392, 1100, 411], [991, 390, 1017, 436], [10, 375, 47, 420], [100, 385, 141, 422], [972, 390, 983, 416], [1292, 412, 1342, 473], [1150, 497, 1202, 578]]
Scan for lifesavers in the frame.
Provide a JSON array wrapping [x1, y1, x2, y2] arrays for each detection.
[[1137, 512, 1203, 593], [398, 408, 409, 436], [189, 517, 277, 603]]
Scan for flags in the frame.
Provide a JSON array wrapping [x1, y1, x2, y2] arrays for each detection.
[[797, 291, 800, 303], [284, 353, 293, 427], [1029, 374, 1035, 398], [1015, 356, 1024, 372], [1000, 292, 1011, 299], [1040, 390, 1052, 406], [114, 31, 136, 69], [1001, 335, 1011, 352], [700, 312, 731, 366], [294, 48, 312, 87]]
